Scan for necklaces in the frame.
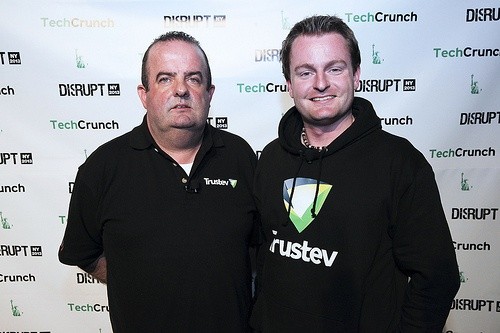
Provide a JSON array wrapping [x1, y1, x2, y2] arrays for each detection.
[[301, 127, 330, 152]]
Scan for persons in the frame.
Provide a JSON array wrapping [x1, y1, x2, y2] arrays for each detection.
[[251, 15, 461, 333], [58, 32, 257, 333]]
